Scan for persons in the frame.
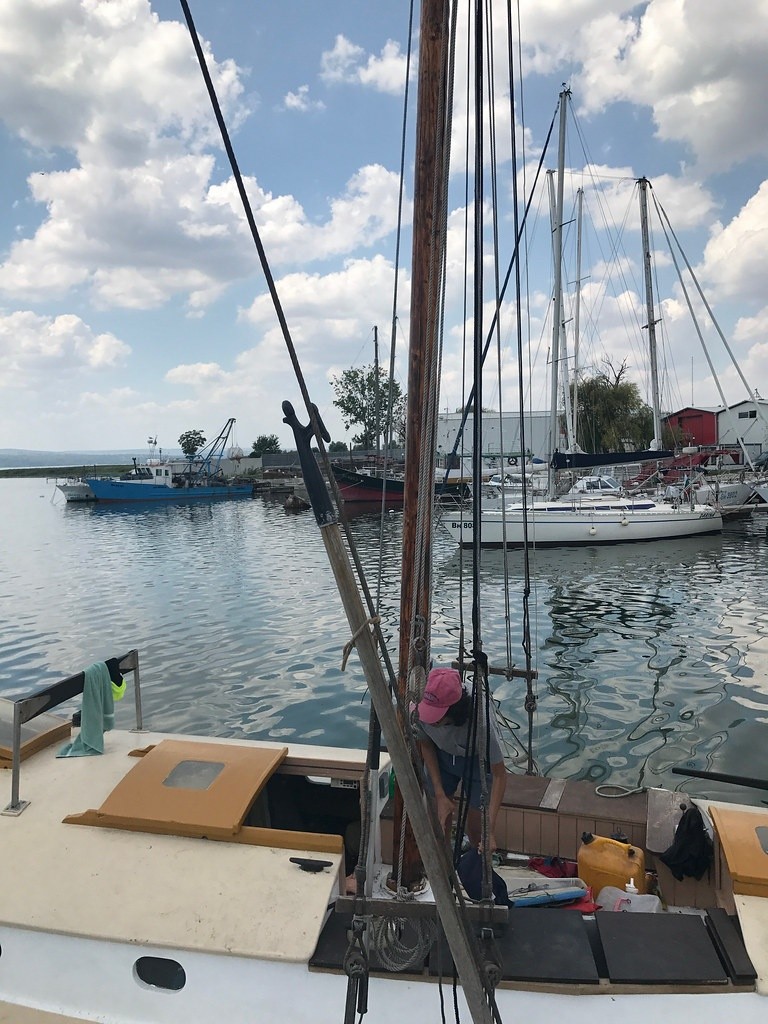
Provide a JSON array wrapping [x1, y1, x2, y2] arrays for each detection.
[[401, 665, 507, 865]]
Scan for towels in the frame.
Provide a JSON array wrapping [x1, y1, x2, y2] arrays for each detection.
[[55, 661, 115, 758]]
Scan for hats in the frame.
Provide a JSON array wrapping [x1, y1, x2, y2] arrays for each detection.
[[418, 667, 463, 724]]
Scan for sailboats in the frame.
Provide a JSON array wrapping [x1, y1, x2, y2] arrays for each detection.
[[4, 0, 767, 1024], [435, 82, 723, 547]]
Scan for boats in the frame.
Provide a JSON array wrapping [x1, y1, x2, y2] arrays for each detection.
[[54, 175, 768, 517]]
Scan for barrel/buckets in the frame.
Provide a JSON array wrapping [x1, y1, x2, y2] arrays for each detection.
[[578, 831, 645, 901]]
